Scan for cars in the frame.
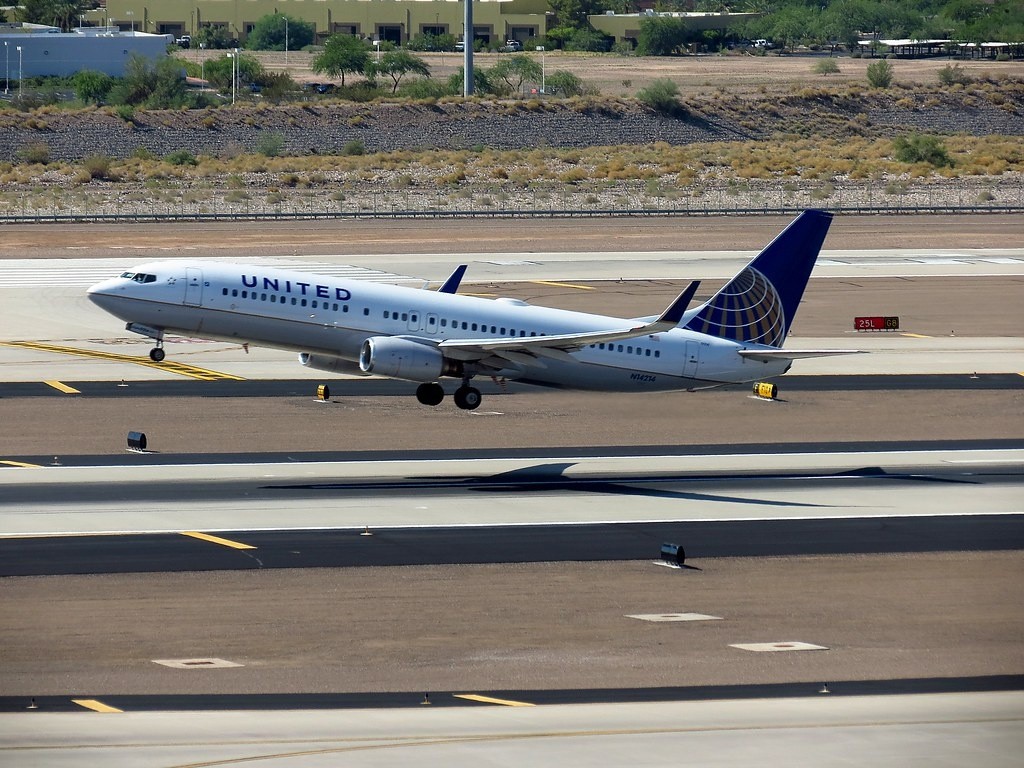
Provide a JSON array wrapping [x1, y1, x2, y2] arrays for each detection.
[[751, 39, 776, 50], [249, 83, 261, 92], [455, 42, 464, 51], [510, 42, 522, 46], [507, 40, 515, 46], [303, 82, 320, 89], [317, 84, 340, 94]]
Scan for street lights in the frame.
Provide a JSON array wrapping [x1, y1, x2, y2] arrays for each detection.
[[4, 41, 10, 95], [200, 42, 207, 90], [16, 42, 26, 99], [191, 11, 194, 36], [126, 8, 134, 36], [104, 8, 108, 32], [282, 16, 289, 66], [235, 48, 242, 93], [226, 52, 236, 105], [436, 12, 439, 36]]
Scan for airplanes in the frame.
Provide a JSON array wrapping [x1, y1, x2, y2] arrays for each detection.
[[85, 208, 836, 410]]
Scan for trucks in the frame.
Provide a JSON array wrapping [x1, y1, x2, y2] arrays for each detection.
[[175, 35, 191, 44]]
